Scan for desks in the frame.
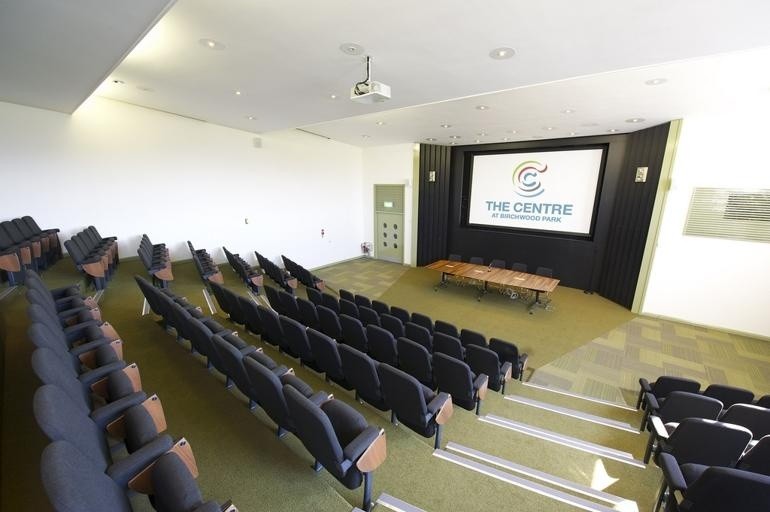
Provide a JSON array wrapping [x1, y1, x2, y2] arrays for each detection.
[[427, 260, 561, 315]]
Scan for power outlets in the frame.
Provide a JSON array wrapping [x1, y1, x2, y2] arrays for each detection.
[[137, 234, 174, 288]]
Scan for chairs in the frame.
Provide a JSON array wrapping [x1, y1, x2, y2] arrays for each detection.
[[64, 225, 120, 292], [255, 252, 298, 295], [0, 215, 60, 287], [306, 286, 512, 395], [205, 278, 455, 449], [265, 284, 488, 415], [223, 246, 263, 295], [136, 275, 387, 507], [281, 255, 326, 292], [188, 241, 225, 286], [340, 289, 528, 380], [25, 269, 240, 508], [637, 375, 767, 508]]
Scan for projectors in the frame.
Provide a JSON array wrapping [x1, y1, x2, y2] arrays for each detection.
[[350, 80, 391, 105]]
